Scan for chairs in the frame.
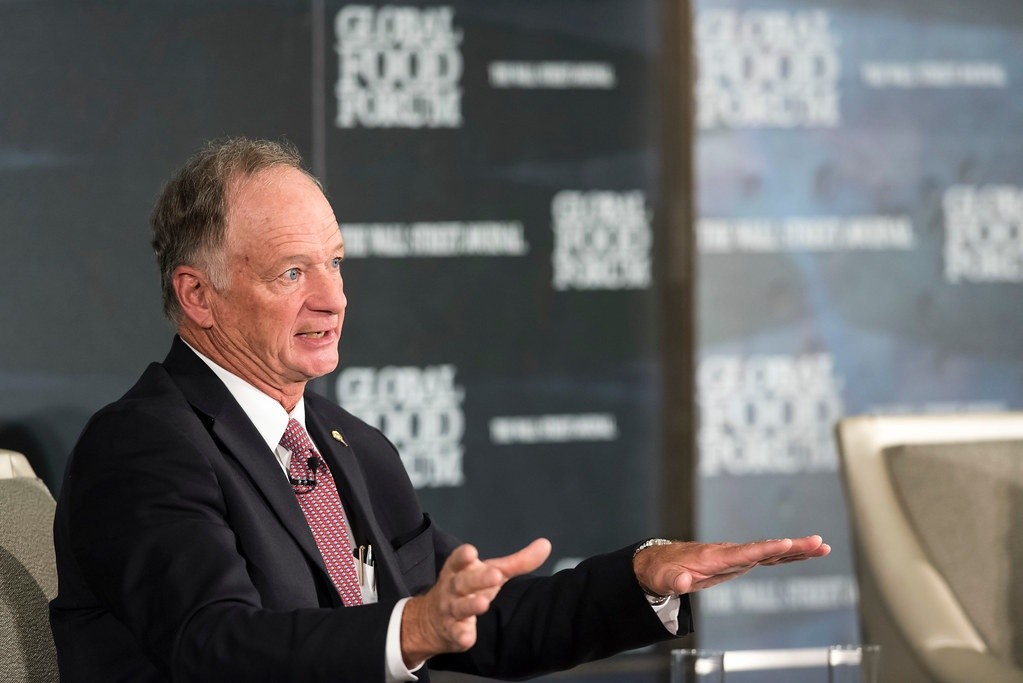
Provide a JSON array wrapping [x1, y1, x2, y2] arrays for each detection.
[[0, 449, 61, 683]]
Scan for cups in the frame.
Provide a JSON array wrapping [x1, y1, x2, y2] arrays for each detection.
[[827, 644, 881, 683], [669, 648, 725, 683]]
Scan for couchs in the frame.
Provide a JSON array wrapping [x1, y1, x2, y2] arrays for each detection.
[[830, 404, 1023, 682]]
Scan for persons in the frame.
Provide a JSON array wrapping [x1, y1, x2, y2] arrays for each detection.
[[53, 134, 831, 683]]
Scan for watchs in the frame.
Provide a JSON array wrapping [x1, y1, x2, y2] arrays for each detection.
[[632, 538, 670, 606]]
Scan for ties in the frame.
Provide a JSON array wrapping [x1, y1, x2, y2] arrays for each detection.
[[280, 418, 363, 607]]
